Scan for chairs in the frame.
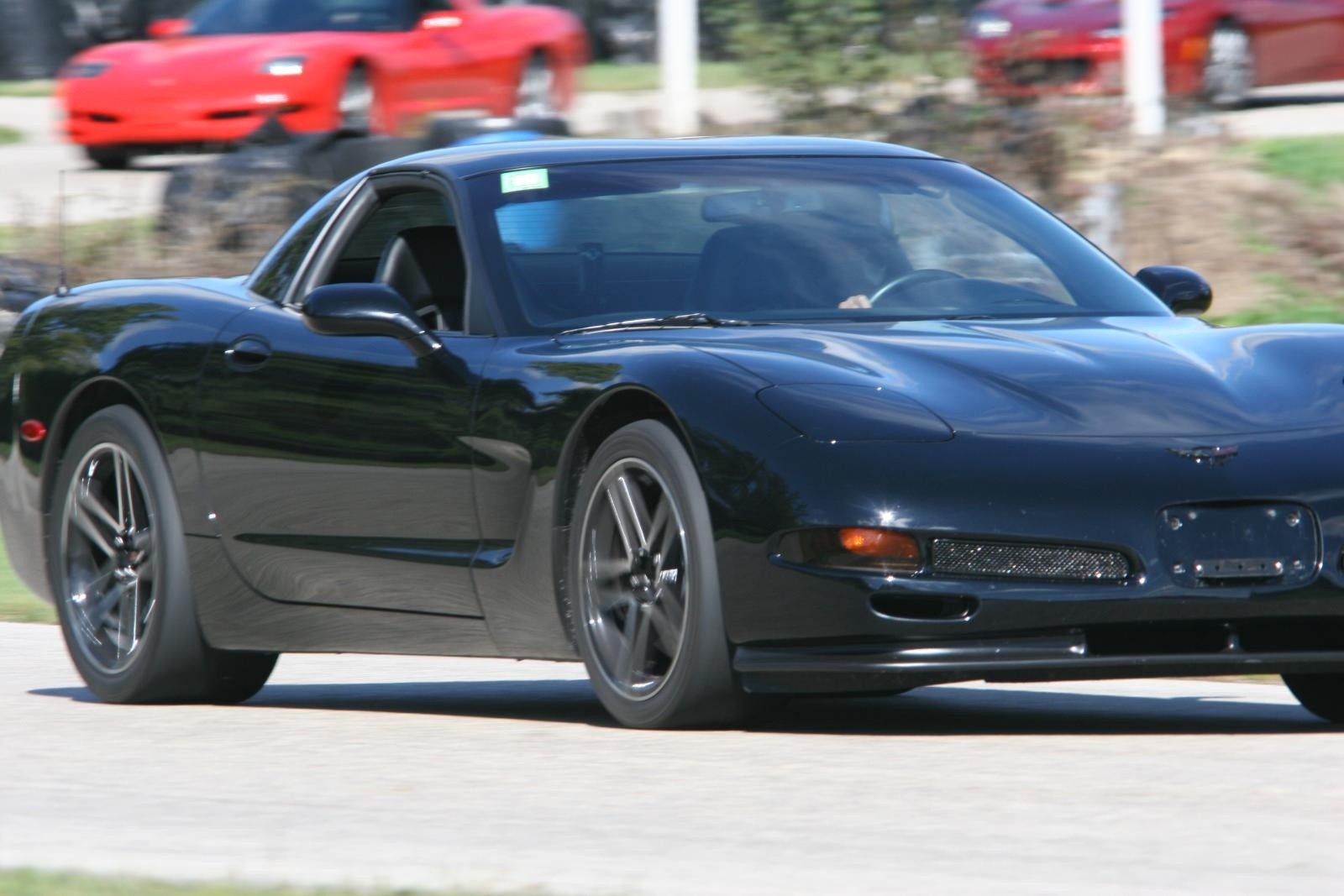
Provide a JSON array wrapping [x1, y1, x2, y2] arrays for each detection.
[[372, 227, 464, 334], [695, 221, 835, 310]]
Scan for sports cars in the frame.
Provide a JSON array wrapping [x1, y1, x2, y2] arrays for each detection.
[[962, 1, 1344, 121], [0, 137, 1343, 729], [51, 0, 592, 171]]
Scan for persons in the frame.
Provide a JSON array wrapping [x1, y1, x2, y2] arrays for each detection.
[[769, 186, 997, 310]]
